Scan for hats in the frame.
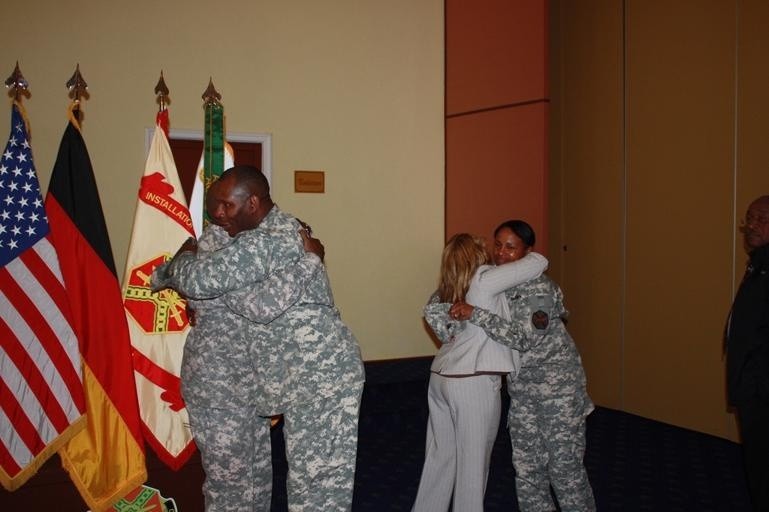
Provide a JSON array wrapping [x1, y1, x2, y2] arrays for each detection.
[[421, 301, 466, 343]]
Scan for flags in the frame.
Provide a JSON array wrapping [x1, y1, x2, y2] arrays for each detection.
[[44, 61, 147, 512], [188, 77, 235, 243], [121, 67, 199, 474], [0, 58, 88, 492]]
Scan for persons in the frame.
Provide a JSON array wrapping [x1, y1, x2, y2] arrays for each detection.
[[421, 220, 597, 512], [149, 164, 366, 512], [180, 181, 326, 512], [410, 233, 550, 511], [721, 196, 769, 444]]
